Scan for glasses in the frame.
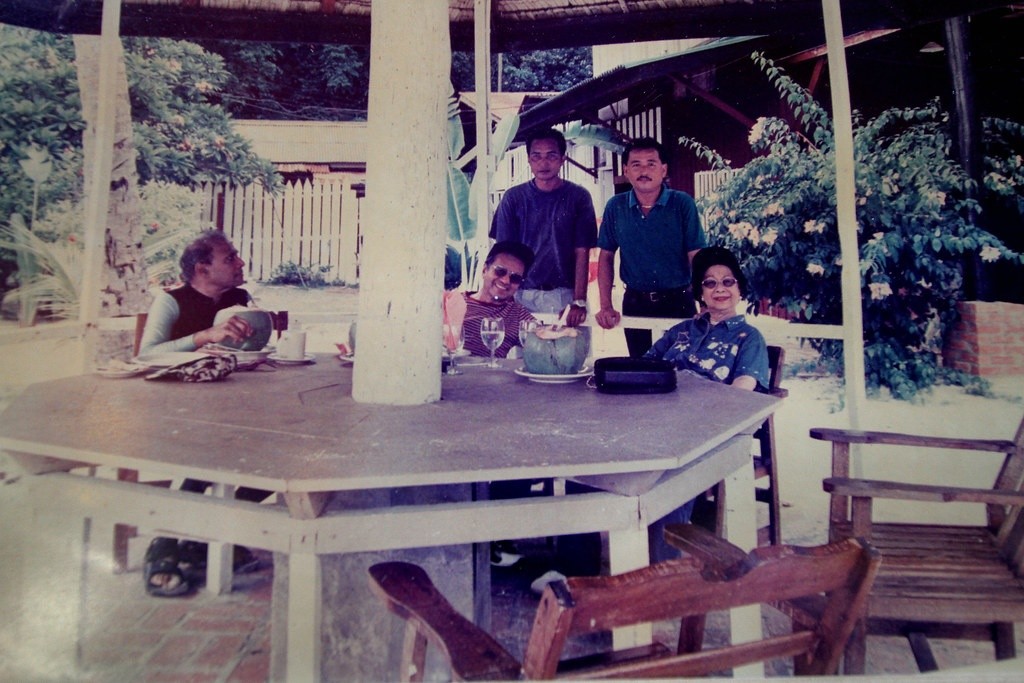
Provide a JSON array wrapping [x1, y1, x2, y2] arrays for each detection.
[[494, 264, 523, 284], [702, 277, 738, 289]]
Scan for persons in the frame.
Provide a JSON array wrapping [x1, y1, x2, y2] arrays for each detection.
[[594, 137, 707, 355], [640, 247, 770, 567], [455, 240, 546, 569], [486, 127, 598, 329], [127, 229, 261, 598]]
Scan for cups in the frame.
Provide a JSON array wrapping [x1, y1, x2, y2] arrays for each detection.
[[277, 330, 305, 358], [518, 320, 543, 348]]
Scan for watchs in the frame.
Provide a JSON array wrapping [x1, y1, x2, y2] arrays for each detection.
[[570, 299, 587, 311]]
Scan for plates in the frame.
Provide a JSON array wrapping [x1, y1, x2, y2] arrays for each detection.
[[514, 365, 594, 384], [339, 355, 353, 363], [440, 350, 473, 363], [268, 353, 315, 365], [212, 351, 275, 362]]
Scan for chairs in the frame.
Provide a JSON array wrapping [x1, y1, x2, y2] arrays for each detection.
[[367, 345, 1024, 683], [111, 311, 291, 573]]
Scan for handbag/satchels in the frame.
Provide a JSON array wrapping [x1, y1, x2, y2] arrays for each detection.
[[641, 290, 680, 312]]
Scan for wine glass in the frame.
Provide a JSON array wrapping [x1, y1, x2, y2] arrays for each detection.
[[481, 318, 505, 369], [441, 324, 465, 374]]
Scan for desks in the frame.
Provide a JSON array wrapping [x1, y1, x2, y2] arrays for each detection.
[[1, 351, 782, 683]]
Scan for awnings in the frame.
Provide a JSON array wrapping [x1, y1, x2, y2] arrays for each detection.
[[493, 35, 776, 178]]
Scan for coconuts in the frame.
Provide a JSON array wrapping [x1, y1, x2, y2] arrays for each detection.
[[523, 325, 587, 375], [213, 304, 272, 351]]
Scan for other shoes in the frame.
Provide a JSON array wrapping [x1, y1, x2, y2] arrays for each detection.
[[491, 552, 525, 567]]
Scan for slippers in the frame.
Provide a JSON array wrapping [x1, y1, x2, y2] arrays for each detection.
[[142, 555, 189, 596], [176, 544, 260, 574]]
